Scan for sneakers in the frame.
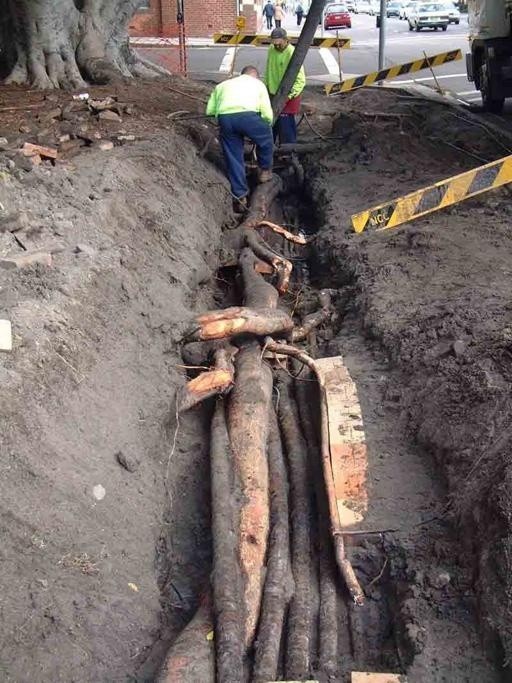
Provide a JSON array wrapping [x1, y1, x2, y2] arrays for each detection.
[[237, 199, 247, 212], [258, 170, 273, 183]]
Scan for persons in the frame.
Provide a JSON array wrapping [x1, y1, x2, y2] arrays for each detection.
[[262, 0, 274, 29], [266, 28, 305, 143], [274, 0, 286, 28], [296, 4, 303, 25], [206, 64, 274, 213]]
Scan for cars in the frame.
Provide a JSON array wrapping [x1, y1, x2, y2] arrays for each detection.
[[324, 2, 351, 29], [347, 0, 460, 31]]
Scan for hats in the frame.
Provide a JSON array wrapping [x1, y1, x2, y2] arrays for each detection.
[[271, 28, 286, 39]]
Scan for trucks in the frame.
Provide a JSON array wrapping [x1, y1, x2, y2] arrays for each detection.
[[466, 0, 512, 110]]
